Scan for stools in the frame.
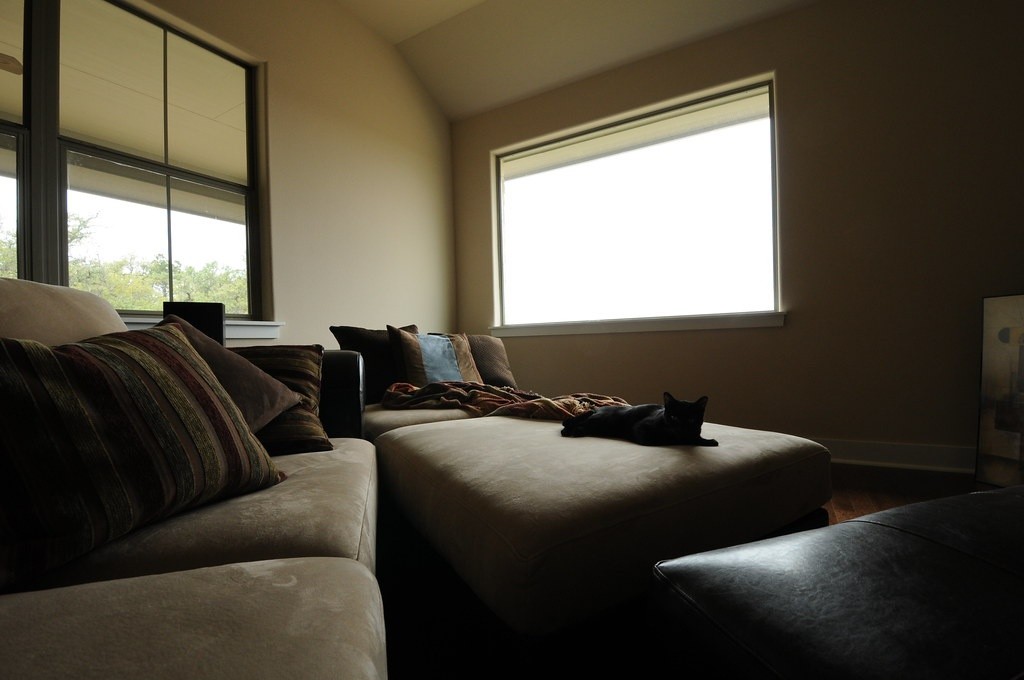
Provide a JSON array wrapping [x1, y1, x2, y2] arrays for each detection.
[[374, 412, 831, 680], [362, 404, 479, 443], [653, 486, 1024, 680]]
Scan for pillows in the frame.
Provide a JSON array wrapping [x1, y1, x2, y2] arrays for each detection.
[[427, 332, 518, 391], [387, 325, 483, 390], [158, 314, 303, 435], [330, 325, 418, 405], [226, 344, 333, 455], [0, 324, 285, 594]]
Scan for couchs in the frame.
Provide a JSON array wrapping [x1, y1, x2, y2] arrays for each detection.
[[0, 277, 388, 680]]
